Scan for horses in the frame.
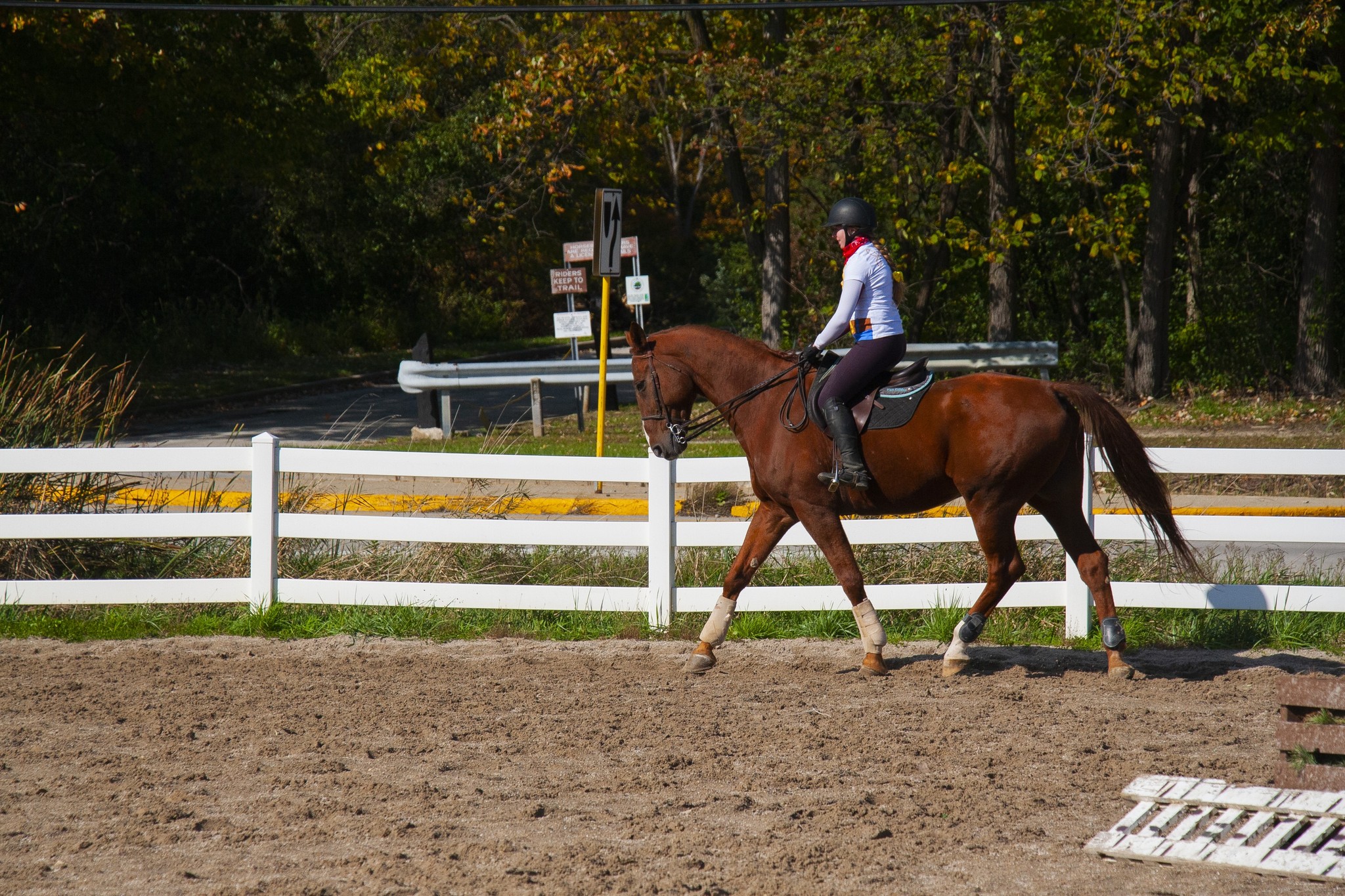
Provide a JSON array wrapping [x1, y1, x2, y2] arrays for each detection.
[[624, 318, 1204, 678]]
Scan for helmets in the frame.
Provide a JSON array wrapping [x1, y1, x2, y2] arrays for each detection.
[[822, 198, 877, 228]]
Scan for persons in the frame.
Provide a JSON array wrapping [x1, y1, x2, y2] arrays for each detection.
[[802, 197, 907, 492]]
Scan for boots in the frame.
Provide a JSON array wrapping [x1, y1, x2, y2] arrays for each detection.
[[817, 396, 868, 491]]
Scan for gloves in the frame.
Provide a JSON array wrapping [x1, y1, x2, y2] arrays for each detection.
[[803, 343, 823, 361]]
